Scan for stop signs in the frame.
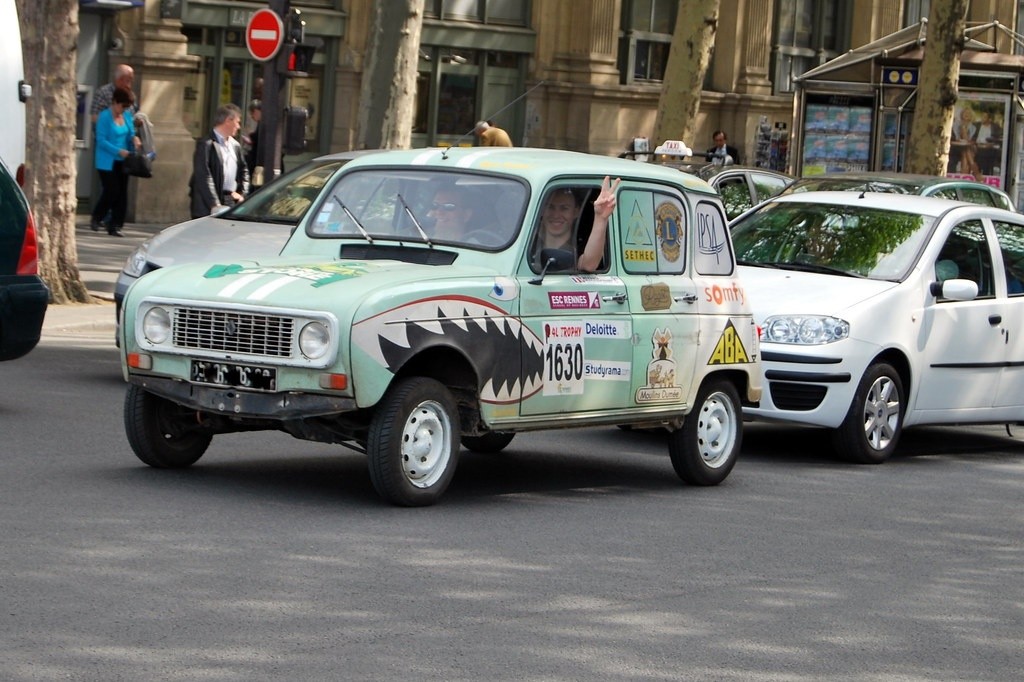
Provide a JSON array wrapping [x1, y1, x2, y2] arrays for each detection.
[[246, 9, 285, 62]]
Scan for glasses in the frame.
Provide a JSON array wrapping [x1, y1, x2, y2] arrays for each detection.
[[122, 104, 131, 109]]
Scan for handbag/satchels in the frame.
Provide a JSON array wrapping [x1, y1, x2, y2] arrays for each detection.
[[125, 147, 152, 178]]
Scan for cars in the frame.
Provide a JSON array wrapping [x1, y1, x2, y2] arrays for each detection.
[[618, 139, 796, 222], [776, 171, 1019, 214], [0, 157, 49, 361], [726, 191, 1024, 464], [117, 147, 764, 509], [113, 148, 432, 347]]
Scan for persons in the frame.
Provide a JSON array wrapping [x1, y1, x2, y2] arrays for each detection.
[[90, 64, 143, 237], [805, 220, 837, 259], [474, 121, 513, 146], [953, 106, 999, 176], [705, 129, 741, 165], [530, 176, 621, 271], [431, 185, 470, 241], [188, 99, 263, 219]]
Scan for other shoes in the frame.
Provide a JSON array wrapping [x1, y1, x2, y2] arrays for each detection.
[[91, 218, 99, 230], [109, 230, 123, 237]]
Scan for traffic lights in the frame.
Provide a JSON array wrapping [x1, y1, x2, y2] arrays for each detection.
[[290, 105, 309, 154], [286, 45, 318, 77]]
